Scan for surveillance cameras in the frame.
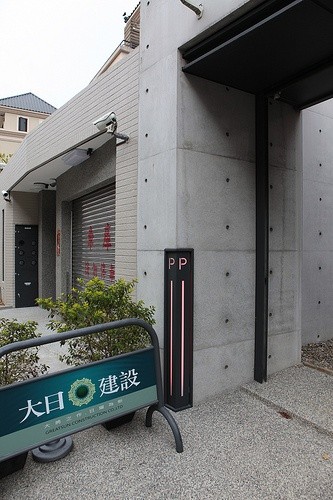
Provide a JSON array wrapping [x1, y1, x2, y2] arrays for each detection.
[[93, 111, 116, 130]]
